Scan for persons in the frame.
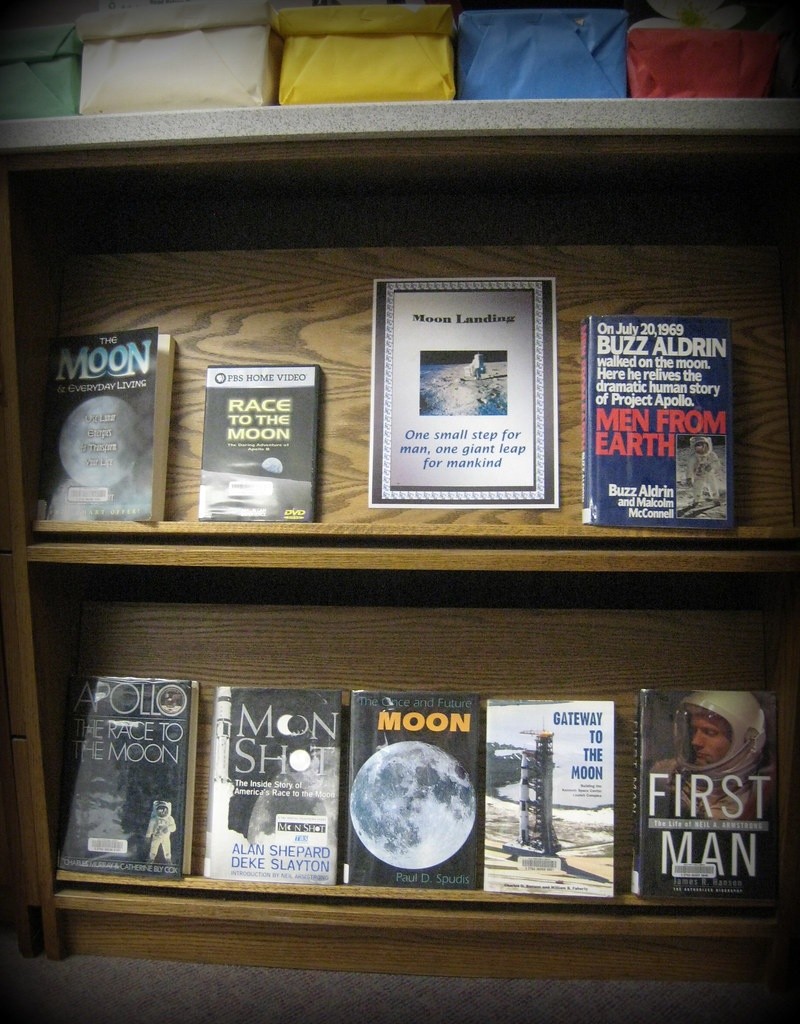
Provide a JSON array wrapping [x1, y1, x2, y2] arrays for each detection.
[[644, 690, 777, 874]]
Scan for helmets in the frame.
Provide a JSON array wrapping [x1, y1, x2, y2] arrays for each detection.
[[156, 801, 170, 819], [677, 690, 765, 789], [692, 437, 712, 458]]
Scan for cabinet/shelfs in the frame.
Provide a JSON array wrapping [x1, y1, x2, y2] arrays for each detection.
[[0, 0, 800, 979]]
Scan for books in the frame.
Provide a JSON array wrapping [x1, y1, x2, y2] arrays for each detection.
[[484, 699, 615, 897], [197, 364, 322, 522], [580, 315, 733, 529], [203, 686, 342, 887], [344, 689, 478, 889], [33, 327, 176, 521], [55, 676, 198, 877], [631, 688, 778, 901]]
